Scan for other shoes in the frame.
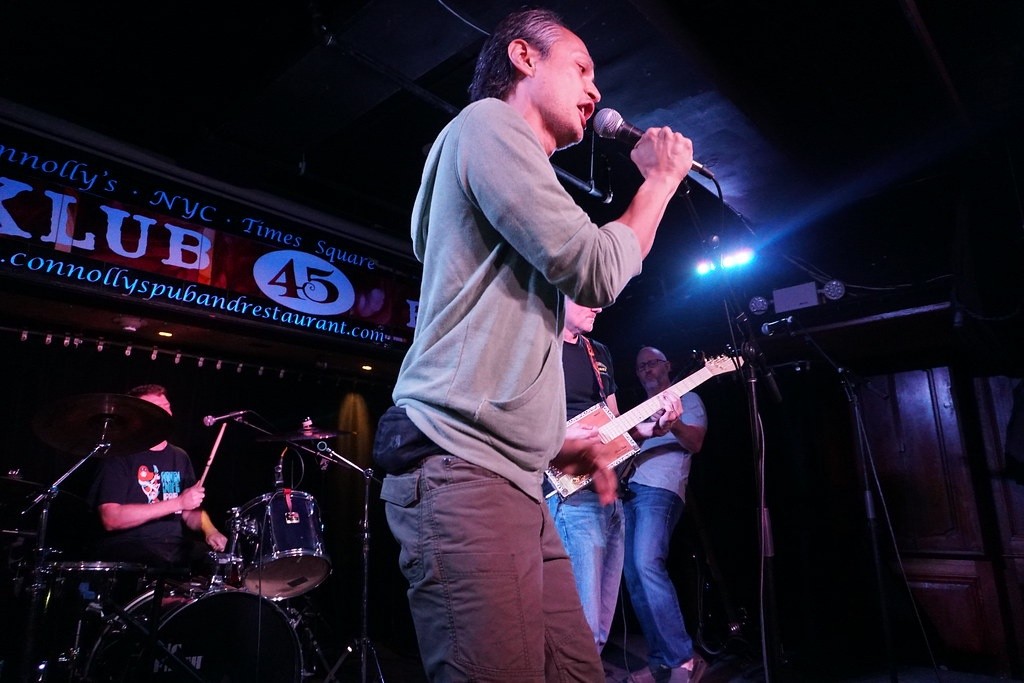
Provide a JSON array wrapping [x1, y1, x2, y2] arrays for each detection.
[[669, 653, 707, 683], [627, 666, 671, 683]]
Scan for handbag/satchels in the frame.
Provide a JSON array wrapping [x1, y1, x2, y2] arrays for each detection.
[[371, 406, 451, 476]]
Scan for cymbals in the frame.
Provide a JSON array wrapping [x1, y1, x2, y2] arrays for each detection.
[[31, 392, 177, 457], [254, 427, 359, 442], [0, 475, 80, 499]]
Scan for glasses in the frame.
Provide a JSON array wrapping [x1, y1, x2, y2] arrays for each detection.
[[635, 359, 668, 373]]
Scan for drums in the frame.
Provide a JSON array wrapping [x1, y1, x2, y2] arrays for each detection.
[[226, 488, 332, 598], [73, 574, 305, 683], [30, 559, 148, 683]]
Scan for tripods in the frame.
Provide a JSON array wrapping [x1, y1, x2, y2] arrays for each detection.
[[291, 440, 385, 683]]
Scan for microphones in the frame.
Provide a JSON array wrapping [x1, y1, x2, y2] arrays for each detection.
[[276, 454, 285, 489], [202, 410, 249, 427], [593, 108, 714, 179], [761, 316, 794, 335]]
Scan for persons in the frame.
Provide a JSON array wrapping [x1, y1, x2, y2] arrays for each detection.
[[622, 345, 708, 681], [97, 383, 228, 577], [373, 7, 693, 682]]
